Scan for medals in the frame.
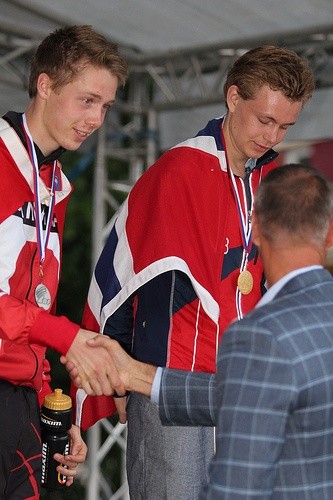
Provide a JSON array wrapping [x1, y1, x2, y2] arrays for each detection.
[[34, 284, 52, 311], [237, 270, 253, 296]]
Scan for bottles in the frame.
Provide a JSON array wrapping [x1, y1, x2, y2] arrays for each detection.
[[40, 388, 73, 491]]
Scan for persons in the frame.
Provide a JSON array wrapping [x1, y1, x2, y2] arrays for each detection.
[[71, 45, 313, 500], [60, 163, 333, 500], [1, 25, 125, 500]]
